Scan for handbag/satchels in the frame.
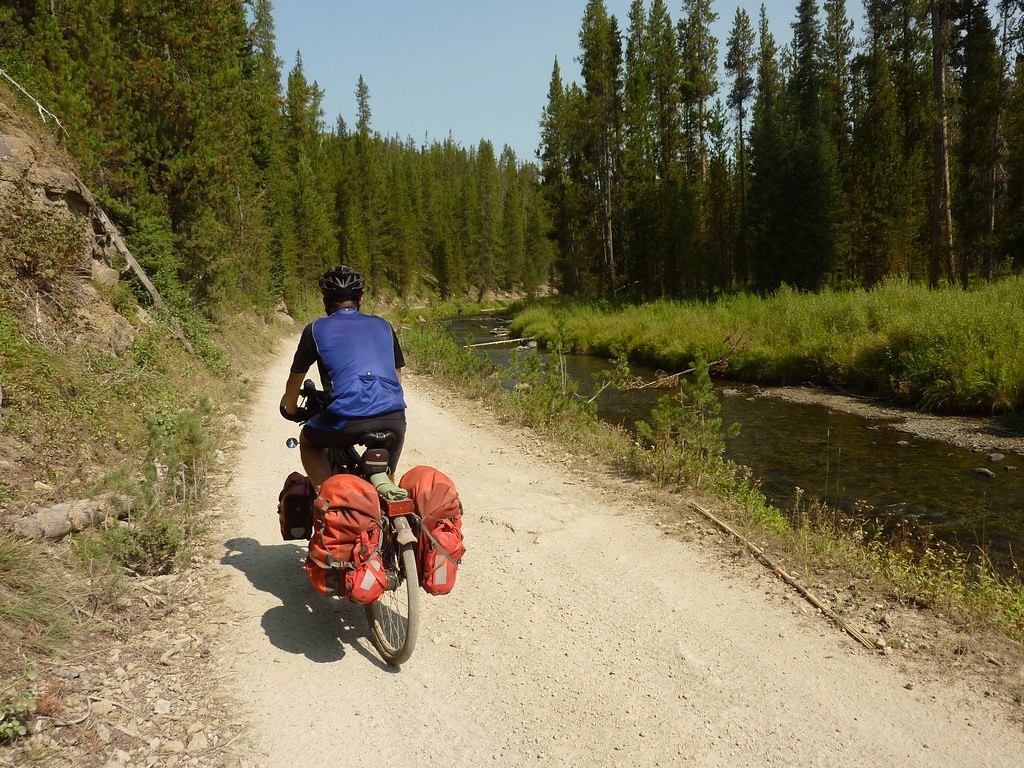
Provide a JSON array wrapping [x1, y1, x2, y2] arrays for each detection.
[[278, 472, 315, 541], [305, 474, 388, 605], [399, 465, 464, 596]]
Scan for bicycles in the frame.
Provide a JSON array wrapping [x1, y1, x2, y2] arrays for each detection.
[[279, 380, 421, 668]]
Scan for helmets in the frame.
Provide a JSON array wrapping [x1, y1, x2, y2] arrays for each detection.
[[319, 265, 365, 298]]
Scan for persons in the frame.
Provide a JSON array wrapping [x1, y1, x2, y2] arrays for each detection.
[[285, 265, 407, 496]]
